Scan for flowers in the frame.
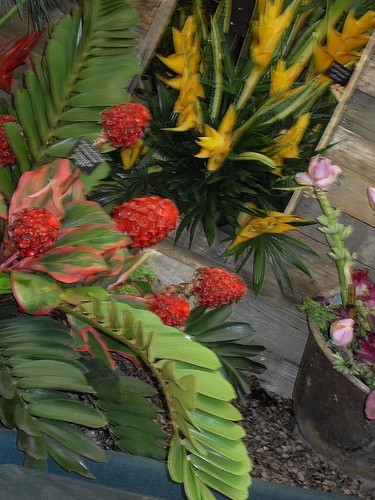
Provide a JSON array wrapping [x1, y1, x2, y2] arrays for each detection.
[[0, 24, 247, 334], [155, 1, 375, 250]]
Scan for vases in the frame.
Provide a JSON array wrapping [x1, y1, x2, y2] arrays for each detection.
[[292, 152, 375, 480]]
